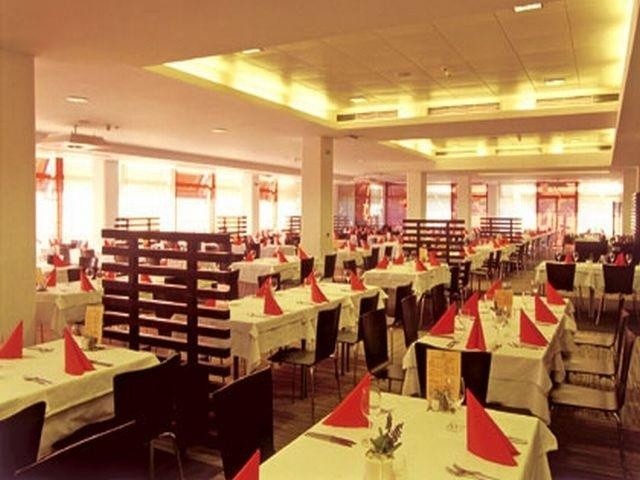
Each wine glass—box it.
[249,250,256,259]
[268,268,352,303]
[359,252,632,480]
[85,267,96,285]
